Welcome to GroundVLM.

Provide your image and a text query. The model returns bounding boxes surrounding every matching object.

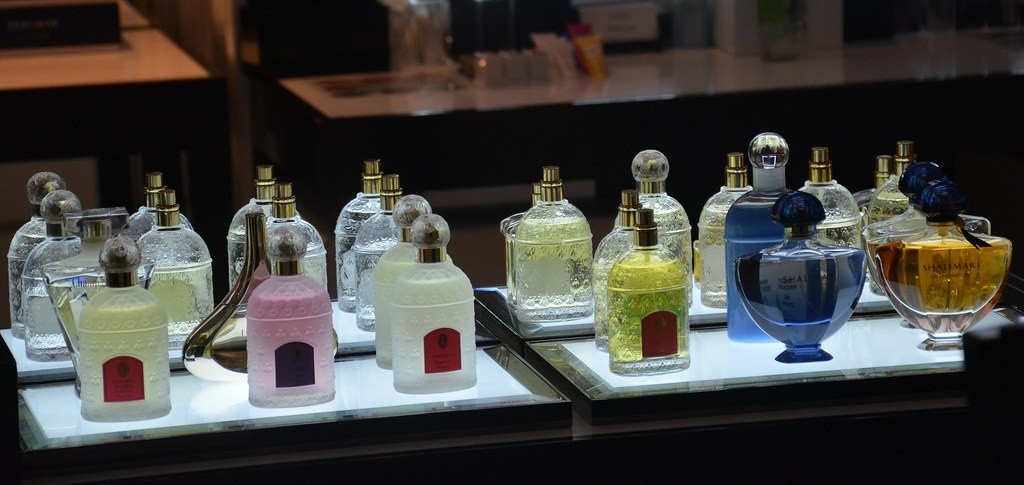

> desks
[0,275,1024,485]
[276,28,1024,234]
[0,0,234,284]
